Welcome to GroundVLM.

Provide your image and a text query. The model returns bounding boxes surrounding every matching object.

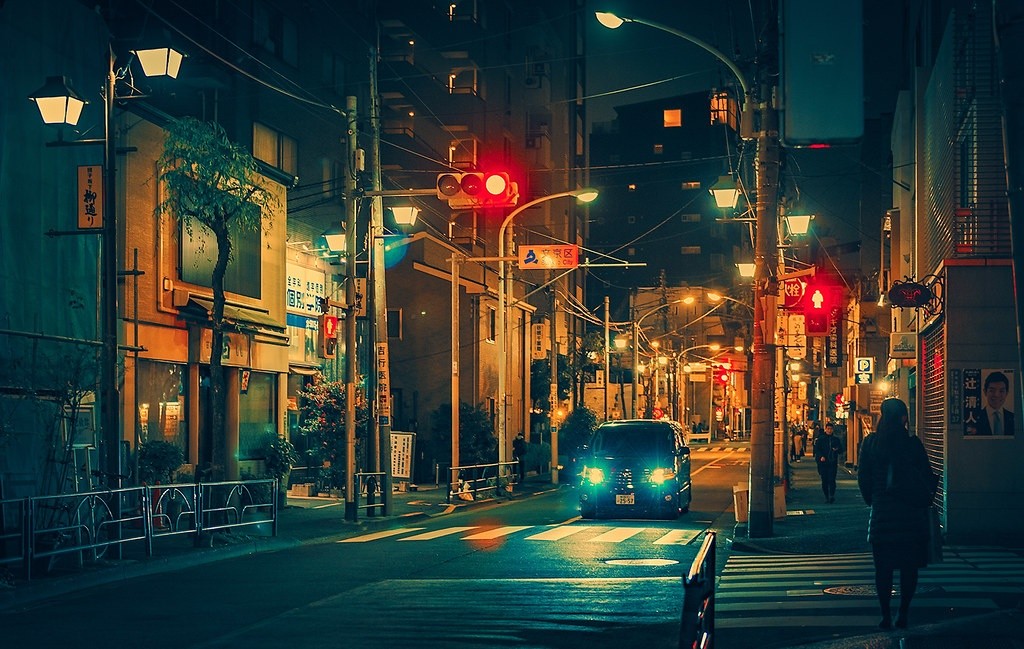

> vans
[578,416,693,518]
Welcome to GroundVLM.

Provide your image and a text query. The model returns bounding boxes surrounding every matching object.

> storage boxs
[733,481,787,521]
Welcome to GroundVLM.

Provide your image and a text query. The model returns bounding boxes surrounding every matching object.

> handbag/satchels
[926,504,943,565]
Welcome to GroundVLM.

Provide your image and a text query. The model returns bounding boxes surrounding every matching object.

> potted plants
[140,439,185,524]
[266,431,298,507]
[242,472,258,513]
[259,472,273,511]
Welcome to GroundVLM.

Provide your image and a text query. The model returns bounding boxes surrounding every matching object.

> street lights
[598,6,815,536]
[23,0,189,565]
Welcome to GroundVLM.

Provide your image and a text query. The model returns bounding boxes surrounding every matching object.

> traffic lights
[316,314,338,359]
[806,284,830,339]
[436,172,518,207]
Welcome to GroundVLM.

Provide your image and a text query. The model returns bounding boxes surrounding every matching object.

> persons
[816,422,843,505]
[691,421,697,442]
[512,432,528,481]
[696,423,703,443]
[980,372,1014,436]
[856,398,939,633]
[787,421,823,463]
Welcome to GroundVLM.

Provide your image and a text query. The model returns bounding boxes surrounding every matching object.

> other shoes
[830,497,835,503]
[895,616,907,627]
[878,617,892,628]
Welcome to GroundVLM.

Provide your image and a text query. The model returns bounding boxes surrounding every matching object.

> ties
[993,411,1003,436]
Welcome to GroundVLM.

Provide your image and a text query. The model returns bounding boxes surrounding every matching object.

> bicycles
[26,471,128,564]
